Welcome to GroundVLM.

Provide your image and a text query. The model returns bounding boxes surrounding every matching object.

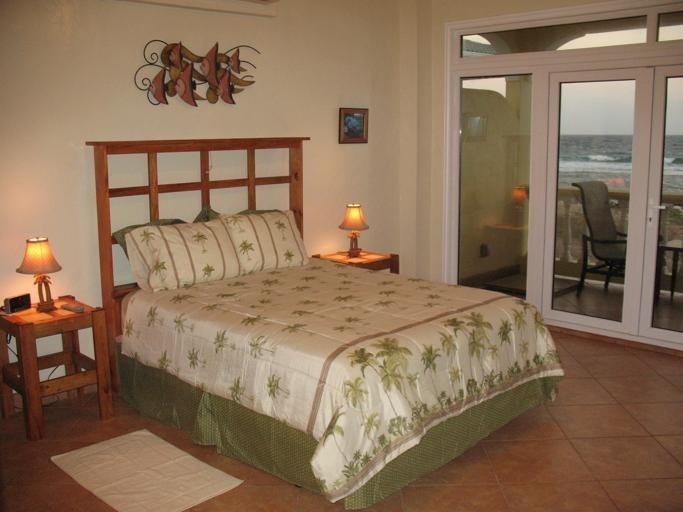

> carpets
[50,428,246,512]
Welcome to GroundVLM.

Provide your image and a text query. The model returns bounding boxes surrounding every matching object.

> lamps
[510,184,527,226]
[339,204,370,258]
[16,236,62,312]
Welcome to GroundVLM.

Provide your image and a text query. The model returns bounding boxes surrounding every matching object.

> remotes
[60,302,84,313]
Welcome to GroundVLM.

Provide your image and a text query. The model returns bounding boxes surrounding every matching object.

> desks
[659,240,683,304]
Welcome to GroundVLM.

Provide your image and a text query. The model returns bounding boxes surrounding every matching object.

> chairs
[113,206,310,295]
[572,181,663,305]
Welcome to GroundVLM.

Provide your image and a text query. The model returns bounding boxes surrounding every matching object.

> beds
[84,137,566,512]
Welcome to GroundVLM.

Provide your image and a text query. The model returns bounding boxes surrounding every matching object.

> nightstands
[312,250,399,275]
[0,295,114,441]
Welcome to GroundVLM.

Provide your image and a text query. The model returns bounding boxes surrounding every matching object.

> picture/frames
[339,107,368,144]
